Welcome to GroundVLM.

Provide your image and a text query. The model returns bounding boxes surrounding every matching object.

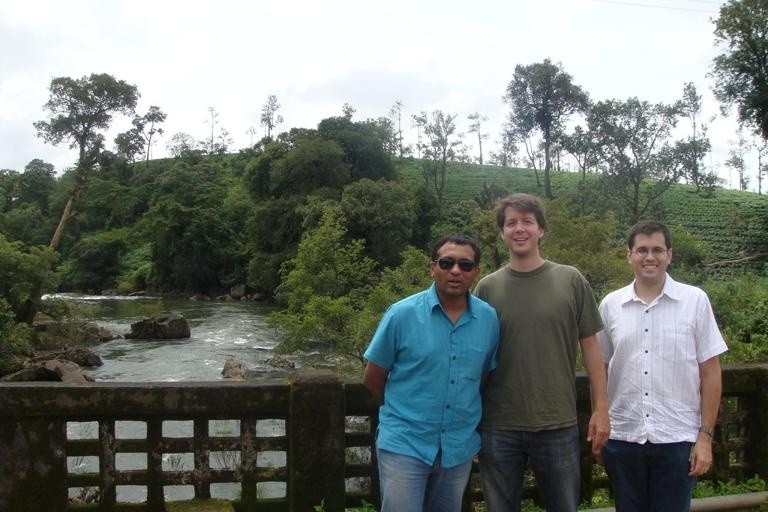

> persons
[590,220,728,510]
[360,235,504,512]
[467,189,612,512]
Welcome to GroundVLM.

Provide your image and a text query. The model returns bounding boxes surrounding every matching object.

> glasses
[632,247,669,255]
[434,257,478,272]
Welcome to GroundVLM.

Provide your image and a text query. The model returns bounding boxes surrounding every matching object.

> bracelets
[698,425,715,437]
